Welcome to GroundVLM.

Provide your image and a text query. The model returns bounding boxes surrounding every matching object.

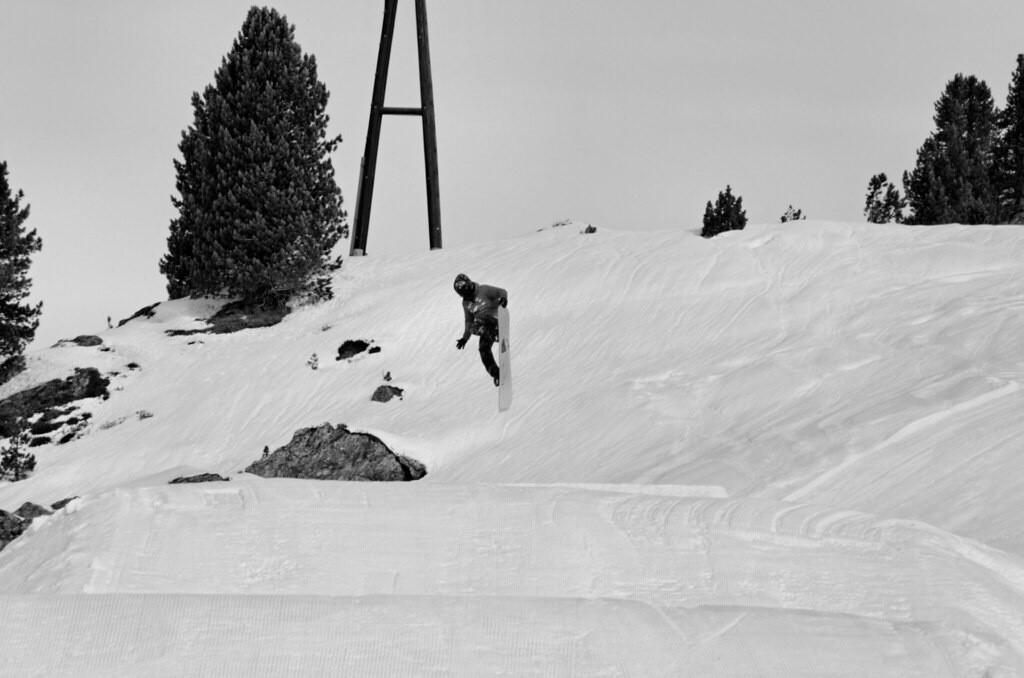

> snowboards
[498,303,514,413]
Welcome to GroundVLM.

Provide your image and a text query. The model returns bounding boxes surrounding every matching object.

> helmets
[454,274,473,298]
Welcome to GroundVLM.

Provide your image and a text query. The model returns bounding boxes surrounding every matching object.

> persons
[454,274,508,386]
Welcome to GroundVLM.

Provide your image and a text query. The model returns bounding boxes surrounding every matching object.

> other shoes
[493,369,499,386]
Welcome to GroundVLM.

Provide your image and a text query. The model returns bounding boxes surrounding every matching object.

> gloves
[456,339,466,349]
[499,297,507,308]
